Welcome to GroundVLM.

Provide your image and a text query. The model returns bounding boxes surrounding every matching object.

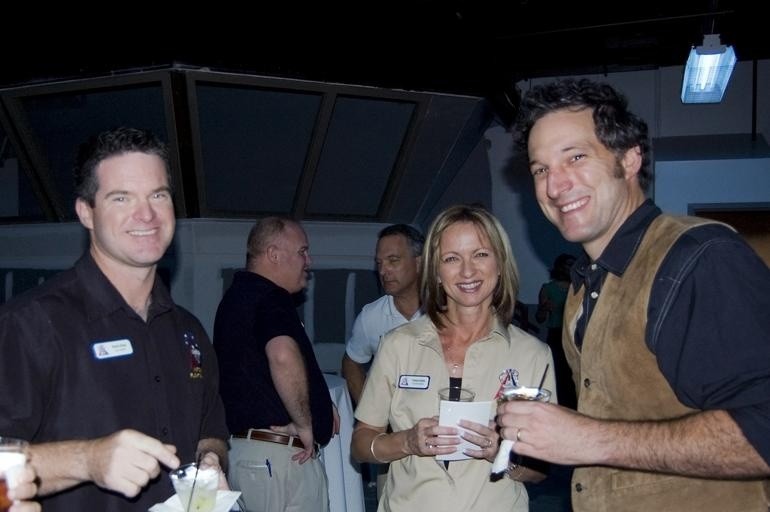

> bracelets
[505,455,521,475]
[370,432,391,464]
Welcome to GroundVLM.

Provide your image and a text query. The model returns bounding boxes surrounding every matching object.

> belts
[232,427,319,456]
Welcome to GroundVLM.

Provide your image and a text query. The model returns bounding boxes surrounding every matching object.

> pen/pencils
[266,458,272,477]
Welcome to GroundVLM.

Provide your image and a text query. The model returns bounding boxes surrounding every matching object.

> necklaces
[443,328,479,373]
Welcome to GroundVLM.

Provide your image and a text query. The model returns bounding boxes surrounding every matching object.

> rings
[517,427,522,441]
[425,438,428,447]
[429,437,432,449]
[488,439,491,448]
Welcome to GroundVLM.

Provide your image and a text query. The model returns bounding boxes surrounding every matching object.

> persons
[535,253,579,412]
[344,205,556,511]
[212,216,341,512]
[0,126,231,512]
[496,76,770,511]
[0,442,43,512]
[340,223,430,505]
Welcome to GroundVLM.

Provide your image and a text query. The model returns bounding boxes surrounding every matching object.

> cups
[498,386,553,406]
[0,435,31,512]
[168,462,221,512]
[436,386,476,418]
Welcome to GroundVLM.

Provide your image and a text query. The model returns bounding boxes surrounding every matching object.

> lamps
[680,34,737,104]
